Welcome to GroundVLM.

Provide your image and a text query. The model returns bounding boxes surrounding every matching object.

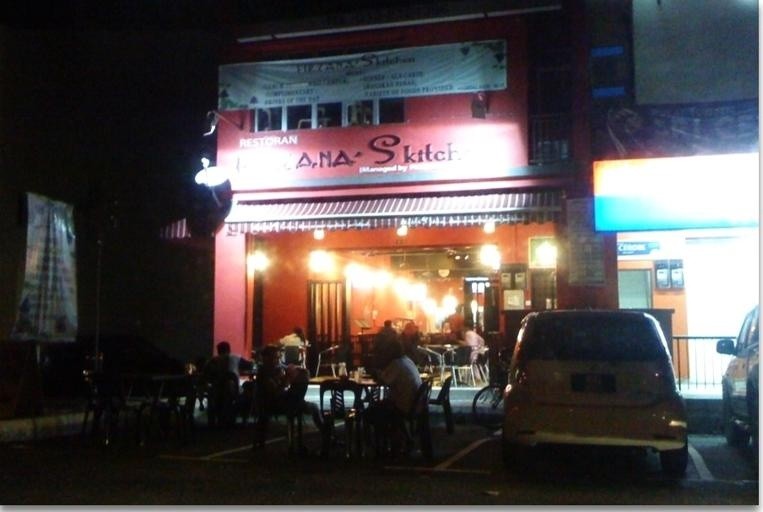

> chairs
[80,348,205,445]
[207,347,310,447]
[319,375,456,457]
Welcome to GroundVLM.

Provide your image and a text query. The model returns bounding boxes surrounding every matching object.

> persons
[377,304,486,369]
[249,343,288,386]
[200,340,253,414]
[278,328,311,364]
[282,344,305,393]
[360,319,425,468]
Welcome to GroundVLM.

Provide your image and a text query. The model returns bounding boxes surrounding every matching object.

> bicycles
[474,331,511,425]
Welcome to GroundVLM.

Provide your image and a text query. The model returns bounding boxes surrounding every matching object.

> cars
[41,333,196,400]
[717,307,759,450]
[499,310,687,471]
